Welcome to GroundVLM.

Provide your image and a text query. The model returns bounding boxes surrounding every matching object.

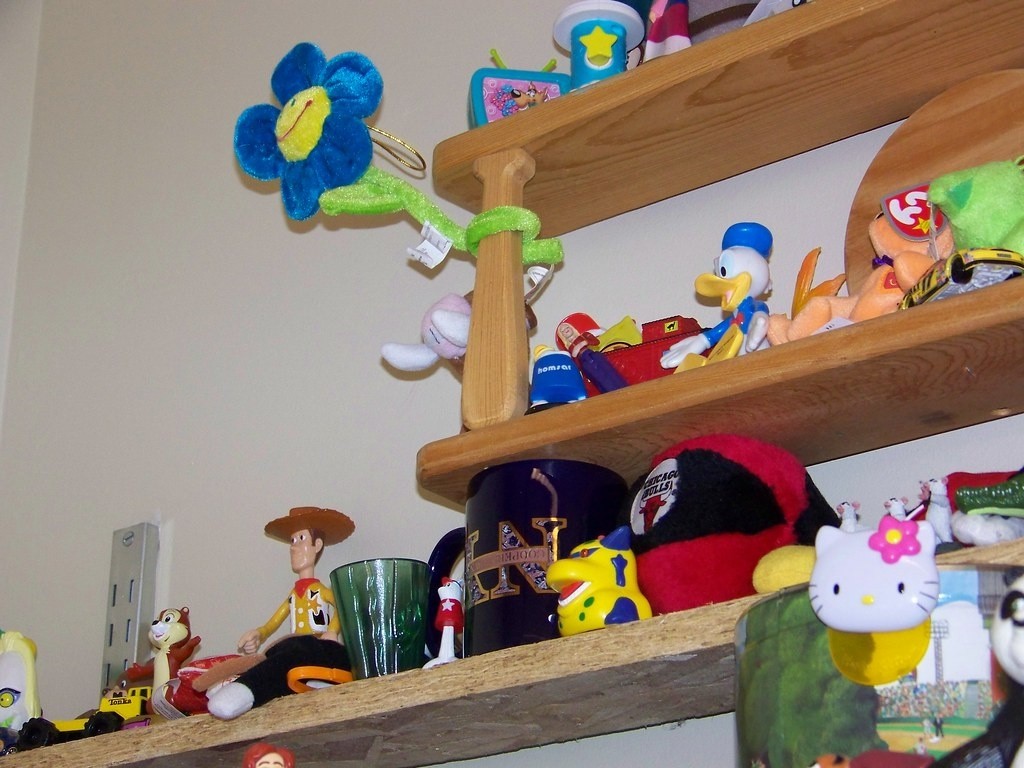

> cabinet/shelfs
[416,0,1024,519]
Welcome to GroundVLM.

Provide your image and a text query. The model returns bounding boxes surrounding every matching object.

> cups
[329,557,437,682]
[423,459,632,660]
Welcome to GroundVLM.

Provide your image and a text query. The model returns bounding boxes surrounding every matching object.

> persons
[209,502,356,718]
[244,742,296,768]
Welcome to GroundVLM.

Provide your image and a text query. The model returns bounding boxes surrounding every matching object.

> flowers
[868,514,921,563]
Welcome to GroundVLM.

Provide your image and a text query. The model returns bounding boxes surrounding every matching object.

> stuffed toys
[639,425,840,619]
[763,159,1024,347]
